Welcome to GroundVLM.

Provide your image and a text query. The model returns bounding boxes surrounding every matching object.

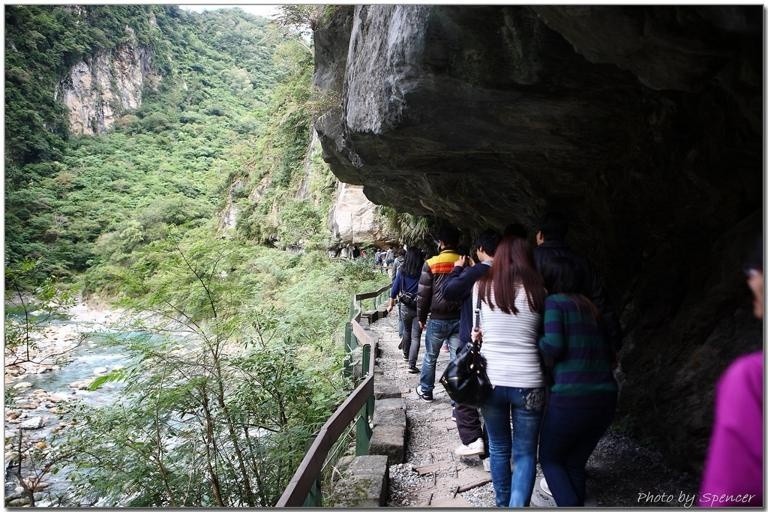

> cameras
[462,256,470,267]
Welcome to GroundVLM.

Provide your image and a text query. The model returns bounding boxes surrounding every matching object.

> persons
[261,218,623,509]
[691,246,763,508]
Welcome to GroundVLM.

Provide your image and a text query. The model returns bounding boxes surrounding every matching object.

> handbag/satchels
[398,291,417,309]
[439,342,493,406]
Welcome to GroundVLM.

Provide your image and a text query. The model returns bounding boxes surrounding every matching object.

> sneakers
[455,438,485,455]
[482,457,491,471]
[403,355,409,361]
[440,344,449,351]
[407,367,420,373]
[415,384,433,403]
